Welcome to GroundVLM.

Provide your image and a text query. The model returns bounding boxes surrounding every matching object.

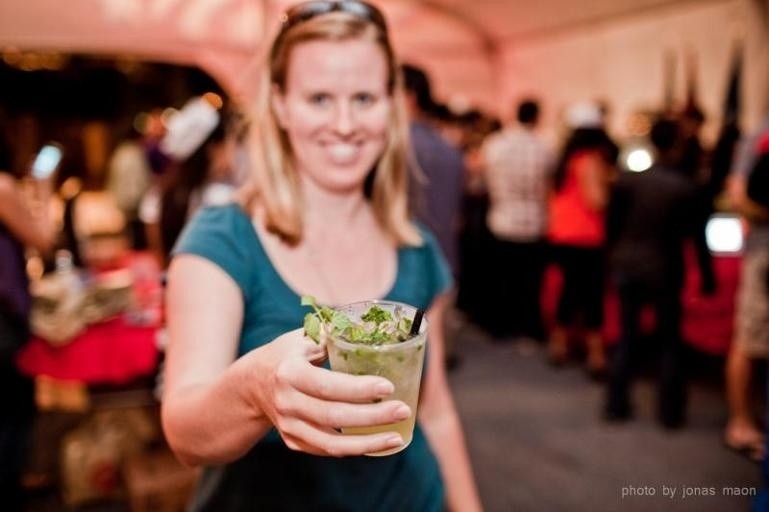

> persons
[2,52,768,510]
[156,3,486,511]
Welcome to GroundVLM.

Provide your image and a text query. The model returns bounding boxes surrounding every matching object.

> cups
[318,298,430,458]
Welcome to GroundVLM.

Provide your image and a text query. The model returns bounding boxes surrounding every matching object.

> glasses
[267,0,391,54]
